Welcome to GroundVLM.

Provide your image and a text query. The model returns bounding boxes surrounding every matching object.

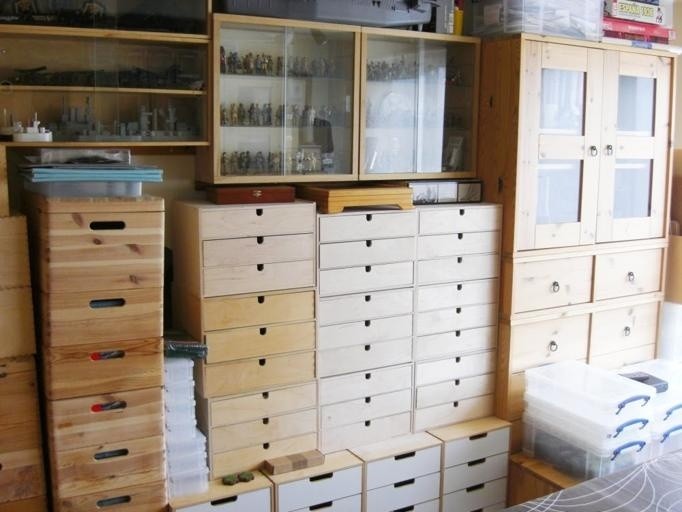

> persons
[221,102,336,127]
[220,46,336,79]
[367,55,463,83]
[222,150,317,175]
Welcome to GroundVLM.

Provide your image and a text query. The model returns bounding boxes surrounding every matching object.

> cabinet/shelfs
[196,11,361,187]
[493,297,664,424]
[0,29,212,147]
[0,0,211,38]
[498,243,669,321]
[358,24,481,182]
[477,30,678,256]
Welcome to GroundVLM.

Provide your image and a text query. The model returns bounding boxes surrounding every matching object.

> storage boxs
[47,386,164,454]
[55,480,168,512]
[180,287,317,398]
[207,187,295,204]
[472,0,602,42]
[440,451,508,493]
[169,473,275,512]
[397,499,439,512]
[293,493,361,512]
[426,415,512,469]
[418,203,504,286]
[51,434,166,498]
[347,432,441,491]
[38,196,166,292]
[521,359,681,483]
[481,502,506,512]
[440,478,507,512]
[260,449,363,512]
[177,202,316,298]
[319,363,414,454]
[45,339,163,399]
[413,276,501,362]
[43,289,169,347]
[316,209,417,297]
[362,473,440,511]
[317,288,414,382]
[197,379,318,481]
[412,349,497,432]
